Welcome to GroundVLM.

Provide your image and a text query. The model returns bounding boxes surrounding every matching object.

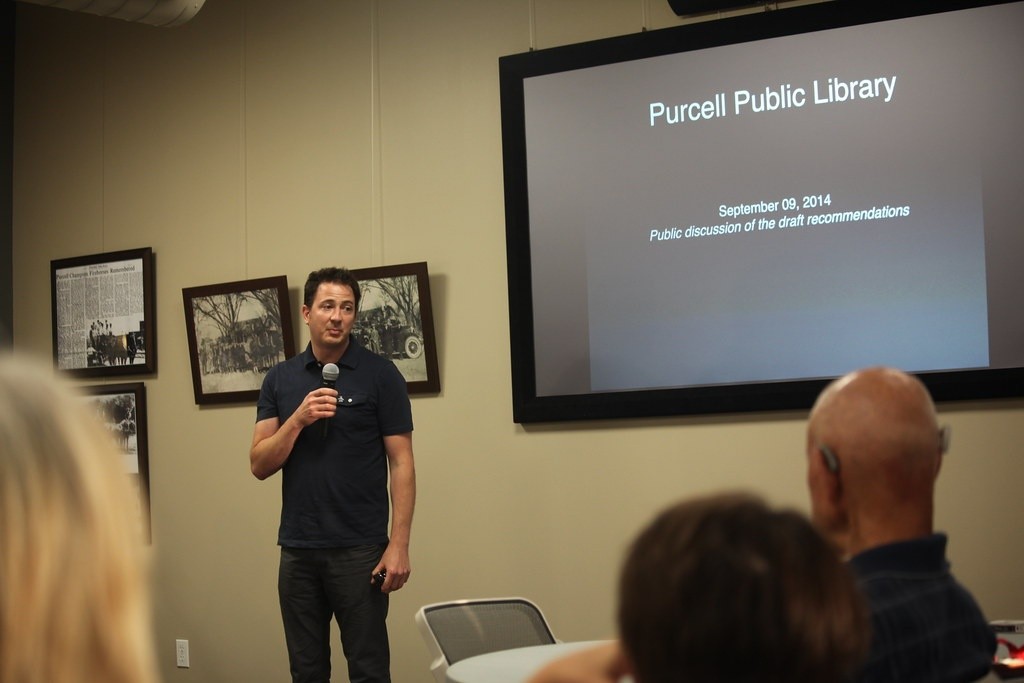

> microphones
[321,363,339,439]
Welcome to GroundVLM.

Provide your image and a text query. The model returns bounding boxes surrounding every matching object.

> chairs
[415,597,563,683]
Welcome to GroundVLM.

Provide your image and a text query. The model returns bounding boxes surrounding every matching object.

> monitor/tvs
[499,0,1024,424]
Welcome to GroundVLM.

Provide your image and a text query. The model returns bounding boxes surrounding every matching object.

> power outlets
[176,639,189,668]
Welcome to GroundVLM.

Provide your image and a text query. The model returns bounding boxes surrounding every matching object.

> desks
[447,639,635,683]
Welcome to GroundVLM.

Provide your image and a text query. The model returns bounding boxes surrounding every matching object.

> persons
[0,350,163,683]
[527,492,873,683]
[248,267,417,683]
[356,319,404,361]
[199,338,226,375]
[807,369,996,683]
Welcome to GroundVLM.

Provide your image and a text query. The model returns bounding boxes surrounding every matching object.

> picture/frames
[350,261,440,394]
[50,247,158,378]
[79,382,152,547]
[183,275,295,404]
